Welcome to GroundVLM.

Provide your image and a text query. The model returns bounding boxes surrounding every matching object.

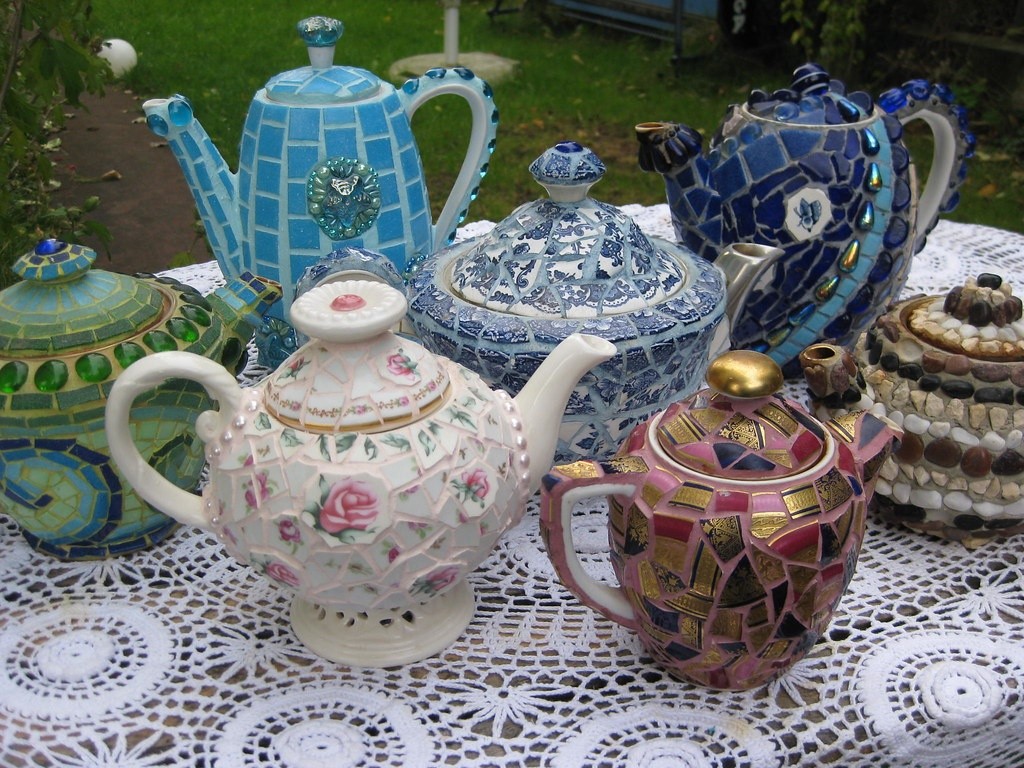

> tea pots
[633,62,974,385]
[799,272,1023,543]
[105,279,619,670]
[0,236,284,563]
[141,15,502,371]
[290,141,787,492]
[539,349,906,693]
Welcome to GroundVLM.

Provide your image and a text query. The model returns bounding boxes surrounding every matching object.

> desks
[0,204,1024,767]
